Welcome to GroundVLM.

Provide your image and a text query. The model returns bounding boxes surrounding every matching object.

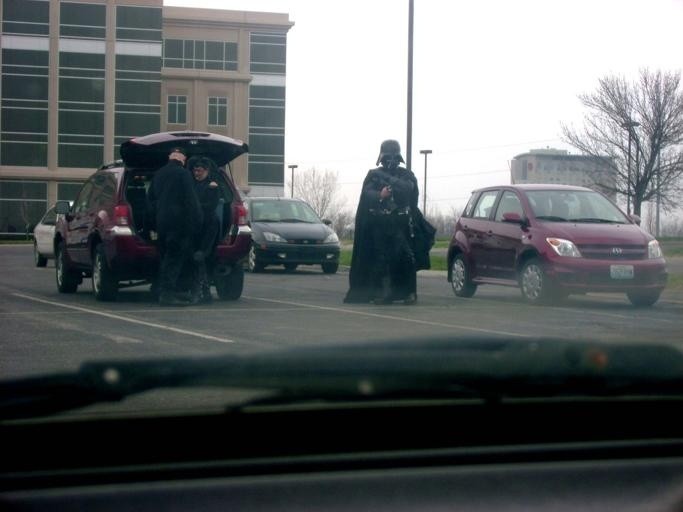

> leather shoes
[158,294,189,306]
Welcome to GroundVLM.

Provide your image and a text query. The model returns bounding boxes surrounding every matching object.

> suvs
[53,131,251,301]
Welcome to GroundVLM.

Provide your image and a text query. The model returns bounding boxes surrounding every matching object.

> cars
[33,200,73,267]
[447,184,669,307]
[241,197,340,273]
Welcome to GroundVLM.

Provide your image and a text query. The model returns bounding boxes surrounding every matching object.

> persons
[146,144,202,307]
[179,154,233,307]
[343,139,436,307]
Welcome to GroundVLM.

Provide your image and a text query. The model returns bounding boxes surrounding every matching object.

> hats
[169,147,186,154]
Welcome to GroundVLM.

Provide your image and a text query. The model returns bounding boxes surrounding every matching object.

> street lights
[417,148,433,221]
[287,163,298,197]
[619,121,640,216]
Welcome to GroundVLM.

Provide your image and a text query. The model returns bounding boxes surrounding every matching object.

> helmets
[376,141,404,165]
